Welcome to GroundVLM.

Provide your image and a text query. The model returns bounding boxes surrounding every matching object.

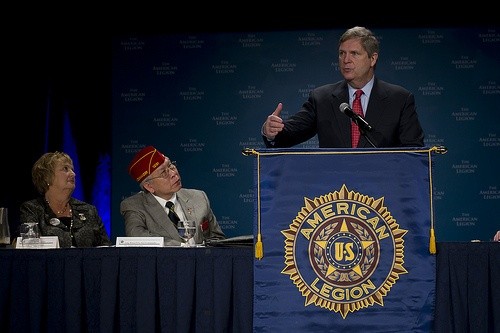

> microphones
[340,103,373,132]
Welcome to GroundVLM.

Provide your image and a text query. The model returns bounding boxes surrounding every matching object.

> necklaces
[48,199,73,237]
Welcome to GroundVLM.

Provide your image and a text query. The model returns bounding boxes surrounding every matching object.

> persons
[491,229,500,242]
[262,27,425,148]
[18,152,113,248]
[120,146,227,246]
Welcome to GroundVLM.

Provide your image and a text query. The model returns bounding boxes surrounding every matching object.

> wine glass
[177,221,196,247]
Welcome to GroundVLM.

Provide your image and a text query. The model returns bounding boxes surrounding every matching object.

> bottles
[0,207,10,248]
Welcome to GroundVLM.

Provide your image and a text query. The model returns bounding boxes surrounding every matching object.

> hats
[127,145,165,186]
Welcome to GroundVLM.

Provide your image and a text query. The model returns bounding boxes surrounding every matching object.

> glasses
[143,161,176,188]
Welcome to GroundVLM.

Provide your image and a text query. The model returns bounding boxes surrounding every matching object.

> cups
[20,223,40,249]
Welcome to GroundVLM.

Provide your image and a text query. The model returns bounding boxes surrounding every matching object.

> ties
[165,201,186,233]
[352,90,365,148]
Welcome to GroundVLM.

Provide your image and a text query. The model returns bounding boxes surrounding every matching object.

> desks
[433,241,500,333]
[0,247,254,333]
